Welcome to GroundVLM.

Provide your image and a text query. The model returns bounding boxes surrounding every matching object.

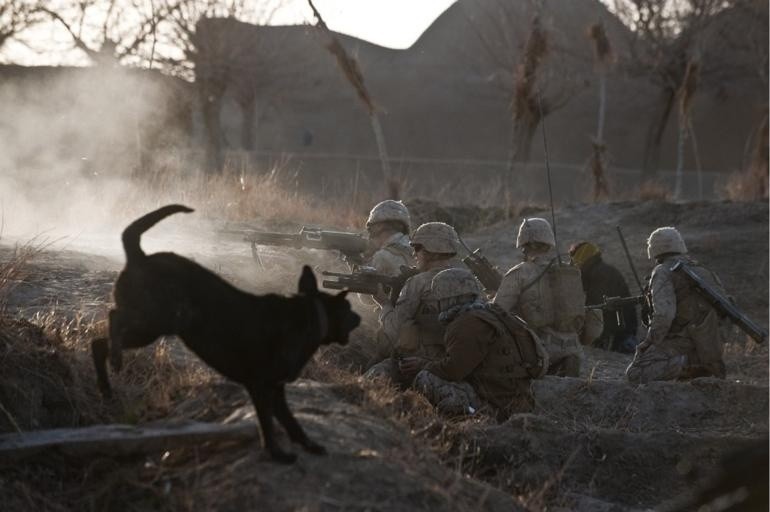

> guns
[219,226,369,272]
[322,264,419,308]
[459,236,503,299]
[585,294,647,326]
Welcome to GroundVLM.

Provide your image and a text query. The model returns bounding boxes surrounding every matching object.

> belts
[538,329,577,347]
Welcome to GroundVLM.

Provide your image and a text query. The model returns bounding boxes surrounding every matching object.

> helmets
[366,199,410,232]
[409,222,458,254]
[516,218,555,249]
[430,267,480,301]
[648,227,689,261]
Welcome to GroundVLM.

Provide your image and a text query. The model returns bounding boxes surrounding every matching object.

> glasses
[414,245,423,252]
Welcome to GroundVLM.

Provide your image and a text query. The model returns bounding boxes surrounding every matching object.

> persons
[567,242,638,354]
[370,222,486,378]
[398,267,535,425]
[624,224,727,386]
[331,198,417,317]
[483,217,587,379]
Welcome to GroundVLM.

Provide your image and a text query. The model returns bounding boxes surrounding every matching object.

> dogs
[88,202,362,465]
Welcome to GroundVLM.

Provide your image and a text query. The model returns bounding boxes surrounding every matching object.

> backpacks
[489,303,549,380]
[520,257,585,332]
[687,268,736,344]
